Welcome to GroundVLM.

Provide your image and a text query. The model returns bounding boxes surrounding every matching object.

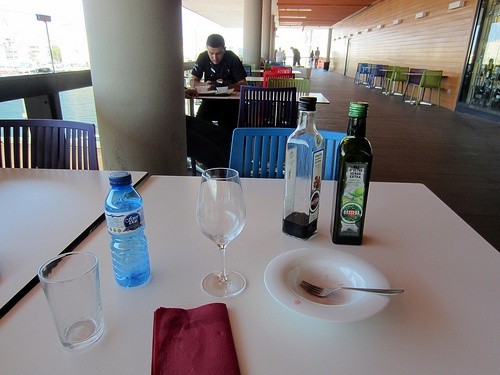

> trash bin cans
[324,62,329,71]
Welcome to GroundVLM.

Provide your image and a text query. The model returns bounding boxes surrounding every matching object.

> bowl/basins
[196,86,209,92]
[216,87,228,92]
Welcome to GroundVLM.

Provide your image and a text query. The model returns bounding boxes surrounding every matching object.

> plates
[198,93,233,96]
[264,248,391,322]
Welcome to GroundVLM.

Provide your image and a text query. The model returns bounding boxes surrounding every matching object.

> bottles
[282,97,325,242]
[330,102,374,246]
[104,172,151,288]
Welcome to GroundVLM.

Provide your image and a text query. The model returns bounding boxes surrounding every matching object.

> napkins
[151,302,241,375]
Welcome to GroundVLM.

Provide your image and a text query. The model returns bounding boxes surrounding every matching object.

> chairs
[226,64,347,181]
[354,61,443,108]
[0,119,99,170]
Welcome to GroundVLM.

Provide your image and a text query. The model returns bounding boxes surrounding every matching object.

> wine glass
[195,167,247,298]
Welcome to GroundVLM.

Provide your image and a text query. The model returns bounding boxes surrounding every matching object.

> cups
[38,252,105,349]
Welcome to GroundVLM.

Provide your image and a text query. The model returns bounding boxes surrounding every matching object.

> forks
[298,281,404,298]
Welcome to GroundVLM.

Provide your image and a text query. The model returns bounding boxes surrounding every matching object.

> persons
[309,50,314,63]
[190,34,248,129]
[273,47,286,66]
[479,59,495,93]
[290,47,301,67]
[315,46,320,69]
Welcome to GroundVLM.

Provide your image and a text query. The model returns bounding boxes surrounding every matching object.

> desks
[0,168,500,375]
[250,69,302,74]
[401,73,448,106]
[379,69,403,95]
[184,86,330,118]
[247,76,303,81]
